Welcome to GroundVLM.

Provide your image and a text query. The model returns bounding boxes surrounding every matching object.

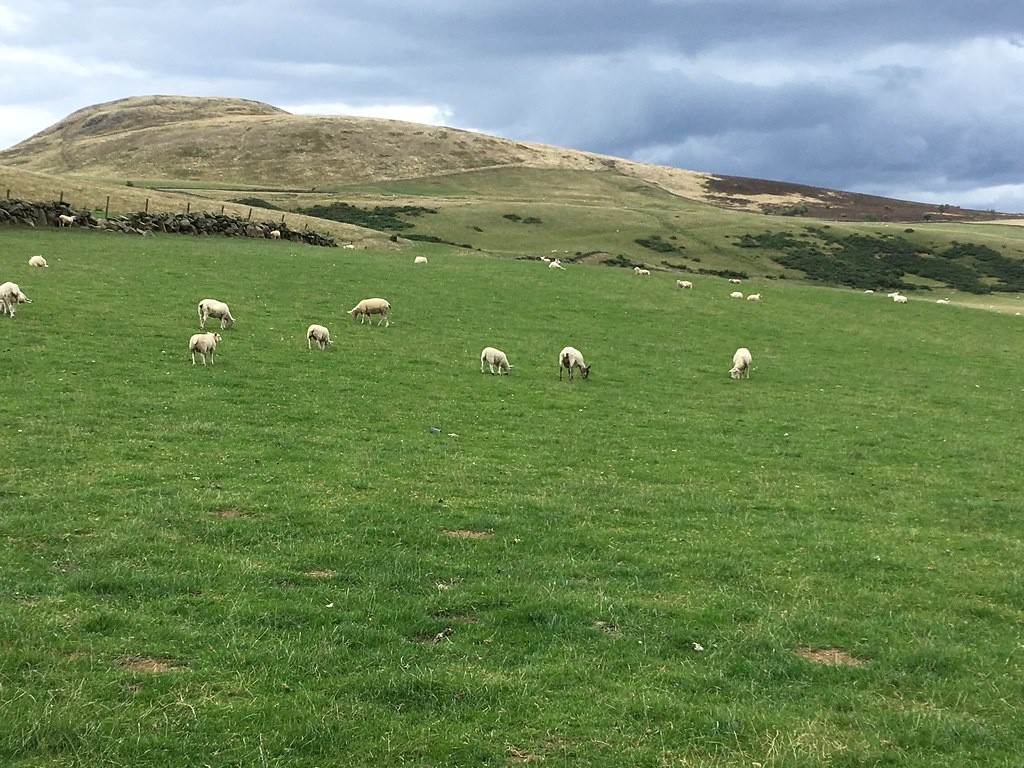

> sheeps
[729,278,741,284]
[58,214,76,228]
[936,297,950,304]
[306,323,333,351]
[28,254,50,268]
[198,298,236,332]
[728,348,752,379]
[189,330,223,365]
[480,346,514,376]
[729,291,743,299]
[347,297,392,329]
[634,266,650,276]
[864,289,875,294]
[746,292,763,301]
[342,244,356,249]
[270,230,281,240]
[676,279,693,290]
[559,346,591,382]
[540,256,566,271]
[0,282,33,319]
[413,256,427,264]
[888,291,908,304]
